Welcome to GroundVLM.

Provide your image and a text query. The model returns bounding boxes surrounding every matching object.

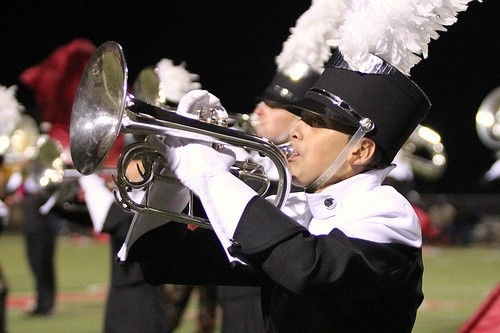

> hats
[249,0,357,107]
[284,1,483,165]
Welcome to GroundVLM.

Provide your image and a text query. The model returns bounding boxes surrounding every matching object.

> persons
[1,42,205,333]
[111,0,483,333]
[194,0,379,333]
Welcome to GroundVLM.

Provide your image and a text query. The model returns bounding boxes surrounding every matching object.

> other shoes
[22,304,55,317]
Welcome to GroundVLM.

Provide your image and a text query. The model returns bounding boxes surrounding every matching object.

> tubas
[69,41,296,231]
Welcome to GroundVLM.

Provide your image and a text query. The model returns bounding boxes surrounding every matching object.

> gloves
[116,87,229,263]
[77,174,115,236]
[163,136,258,267]
